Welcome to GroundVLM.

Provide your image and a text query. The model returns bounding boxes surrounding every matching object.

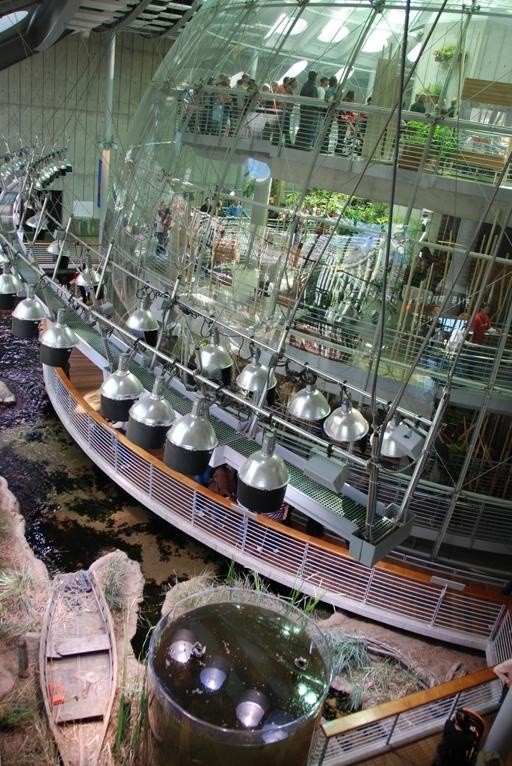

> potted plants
[399,119,446,172]
[414,83,443,104]
[433,45,470,69]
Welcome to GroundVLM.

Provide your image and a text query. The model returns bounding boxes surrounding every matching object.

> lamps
[286,374,331,419]
[235,351,278,391]
[125,288,160,331]
[149,295,178,324]
[370,413,409,459]
[101,352,144,422]
[126,374,176,450]
[164,396,219,475]
[391,418,429,460]
[0,143,114,366]
[236,429,290,514]
[324,393,370,442]
[193,326,234,369]
[304,447,348,493]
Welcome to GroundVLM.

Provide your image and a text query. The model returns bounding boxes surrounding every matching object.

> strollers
[342,114,366,157]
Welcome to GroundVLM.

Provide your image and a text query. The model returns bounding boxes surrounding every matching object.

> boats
[39,569,118,766]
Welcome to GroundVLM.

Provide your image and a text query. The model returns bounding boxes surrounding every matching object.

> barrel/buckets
[143,585,336,766]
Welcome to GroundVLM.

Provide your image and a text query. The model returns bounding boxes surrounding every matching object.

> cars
[461,133,512,178]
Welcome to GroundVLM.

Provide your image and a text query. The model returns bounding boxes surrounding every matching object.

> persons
[200,197,248,218]
[469,303,491,377]
[420,304,442,363]
[403,246,433,288]
[410,95,427,113]
[195,463,290,553]
[435,423,455,461]
[178,71,372,157]
[154,208,173,255]
[448,100,456,117]
